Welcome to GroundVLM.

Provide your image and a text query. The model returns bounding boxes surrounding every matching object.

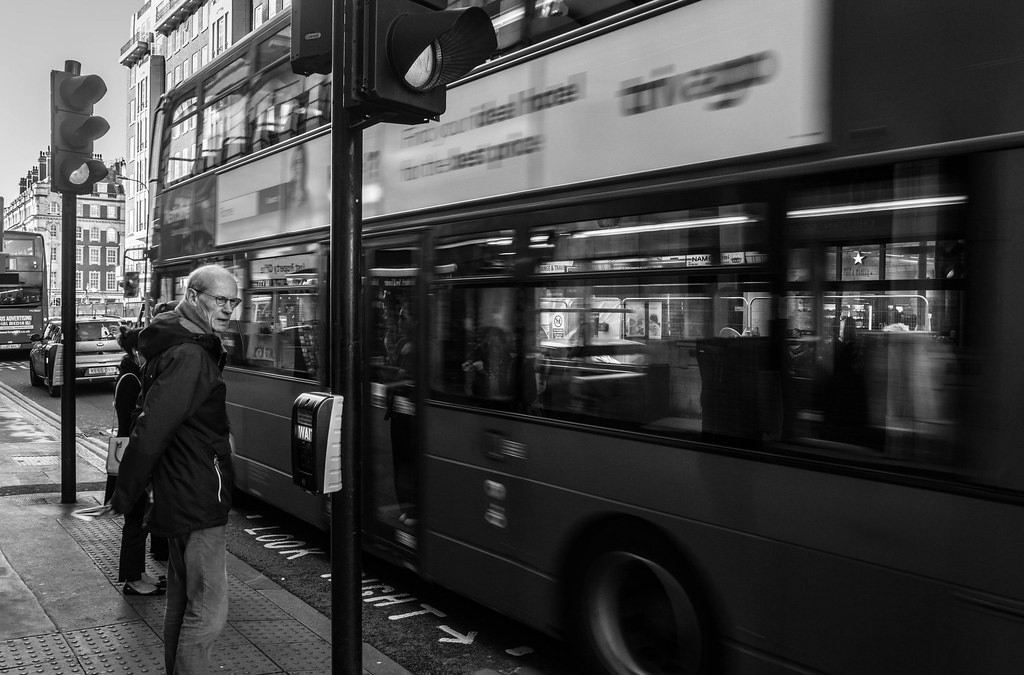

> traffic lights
[359,0,499,116]
[50,70,108,194]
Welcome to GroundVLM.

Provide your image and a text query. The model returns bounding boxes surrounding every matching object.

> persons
[106,264,241,675]
[149,300,181,565]
[102,325,168,597]
[275,146,311,229]
[375,288,515,405]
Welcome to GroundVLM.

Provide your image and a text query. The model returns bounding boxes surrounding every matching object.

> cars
[30,315,132,397]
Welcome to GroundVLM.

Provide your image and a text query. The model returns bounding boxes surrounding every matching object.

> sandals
[124,571,166,595]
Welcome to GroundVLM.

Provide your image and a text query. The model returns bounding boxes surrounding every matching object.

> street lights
[117,177,150,293]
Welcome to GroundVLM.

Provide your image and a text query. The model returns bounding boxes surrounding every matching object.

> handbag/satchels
[106,435,130,475]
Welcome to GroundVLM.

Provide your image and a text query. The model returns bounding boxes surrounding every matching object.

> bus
[119,0,1024,675]
[0,230,50,355]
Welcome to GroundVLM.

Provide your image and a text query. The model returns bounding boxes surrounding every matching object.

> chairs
[284,104,329,139]
[190,149,221,177]
[247,123,285,155]
[220,137,251,165]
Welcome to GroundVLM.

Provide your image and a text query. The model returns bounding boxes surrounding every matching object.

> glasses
[190,285,242,307]
